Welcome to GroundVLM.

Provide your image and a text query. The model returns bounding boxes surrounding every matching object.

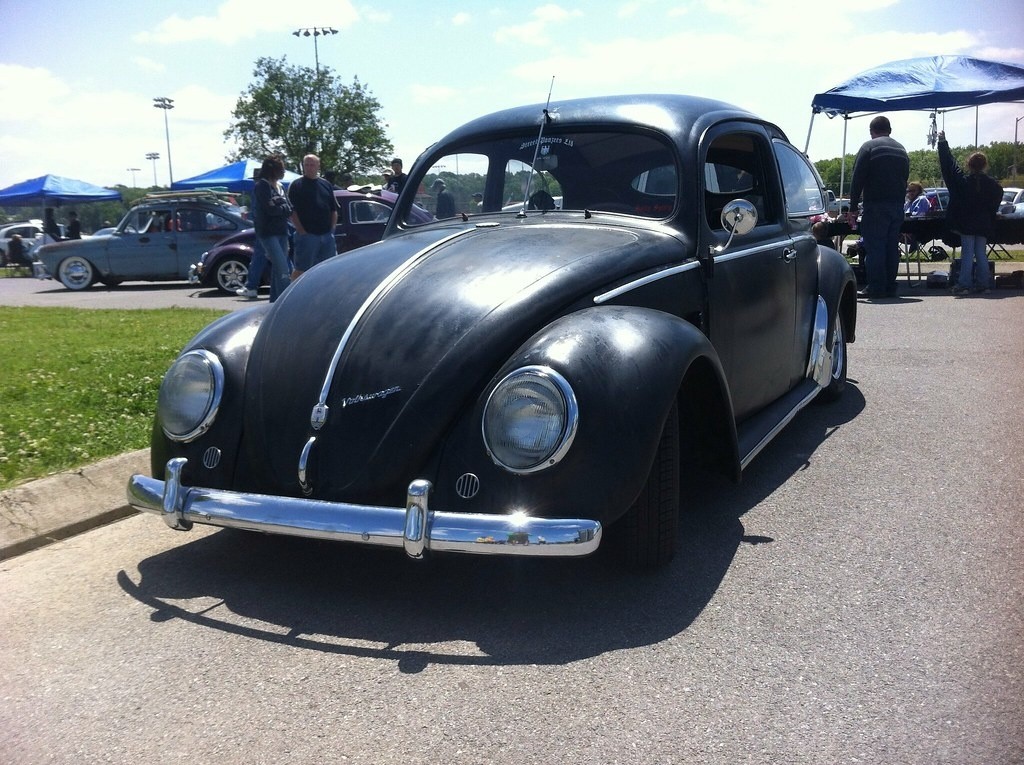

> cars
[923,188,948,215]
[33,197,256,293]
[996,187,1024,220]
[128,93,855,583]
[805,189,850,214]
[189,189,439,296]
[0,222,92,268]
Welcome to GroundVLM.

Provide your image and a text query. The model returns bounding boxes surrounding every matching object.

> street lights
[152,96,175,184]
[145,153,159,186]
[292,26,339,139]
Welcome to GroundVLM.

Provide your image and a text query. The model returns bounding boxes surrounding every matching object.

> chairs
[167,218,183,232]
[6,250,29,278]
[985,205,1016,260]
[896,216,936,261]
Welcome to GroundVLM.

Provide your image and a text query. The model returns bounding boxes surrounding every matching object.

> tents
[803,56,1024,253]
[168,158,303,204]
[0,174,125,244]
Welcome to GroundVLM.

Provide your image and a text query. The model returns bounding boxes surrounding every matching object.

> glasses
[842,213,848,215]
[908,189,918,193]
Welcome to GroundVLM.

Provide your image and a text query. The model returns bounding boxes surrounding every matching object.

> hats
[472,192,483,198]
[431,178,445,189]
[381,168,394,176]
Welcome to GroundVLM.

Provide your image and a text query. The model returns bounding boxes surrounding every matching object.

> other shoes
[235,286,258,298]
[950,285,969,295]
[856,284,886,298]
[974,286,992,294]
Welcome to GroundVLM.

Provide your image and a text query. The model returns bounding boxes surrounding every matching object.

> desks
[813,213,1022,288]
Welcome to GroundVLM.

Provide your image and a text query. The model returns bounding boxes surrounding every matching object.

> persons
[7,234,34,276]
[810,181,931,278]
[65,211,82,240]
[236,154,408,304]
[472,192,484,212]
[148,210,207,232]
[430,179,456,221]
[842,113,908,297]
[936,130,1005,294]
[44,208,61,240]
